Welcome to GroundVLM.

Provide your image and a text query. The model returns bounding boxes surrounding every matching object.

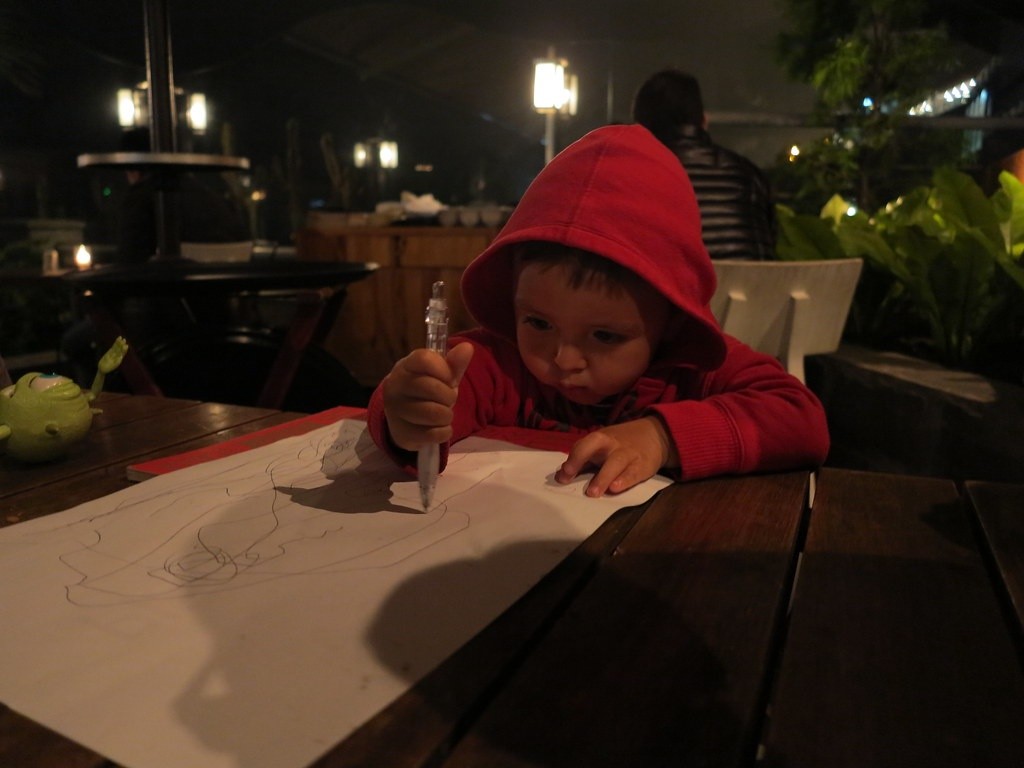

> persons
[366,125,829,497]
[633,71,782,261]
[83,128,235,398]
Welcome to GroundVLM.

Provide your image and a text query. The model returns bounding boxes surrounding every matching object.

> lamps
[114,79,147,133]
[530,45,579,163]
[174,79,209,137]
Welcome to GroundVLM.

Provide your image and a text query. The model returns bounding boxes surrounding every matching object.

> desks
[0,390,1023,768]
[0,257,374,408]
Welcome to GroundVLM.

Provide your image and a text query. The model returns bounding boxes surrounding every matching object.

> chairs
[712,256,863,508]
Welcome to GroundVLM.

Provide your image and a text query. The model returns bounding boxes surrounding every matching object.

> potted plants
[771,160,1023,482]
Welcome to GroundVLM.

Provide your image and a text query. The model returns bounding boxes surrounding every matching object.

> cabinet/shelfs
[296,224,497,387]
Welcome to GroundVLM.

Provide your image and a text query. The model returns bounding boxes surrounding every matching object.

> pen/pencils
[415,280,448,511]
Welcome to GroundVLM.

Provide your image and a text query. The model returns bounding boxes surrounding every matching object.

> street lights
[533,59,580,168]
